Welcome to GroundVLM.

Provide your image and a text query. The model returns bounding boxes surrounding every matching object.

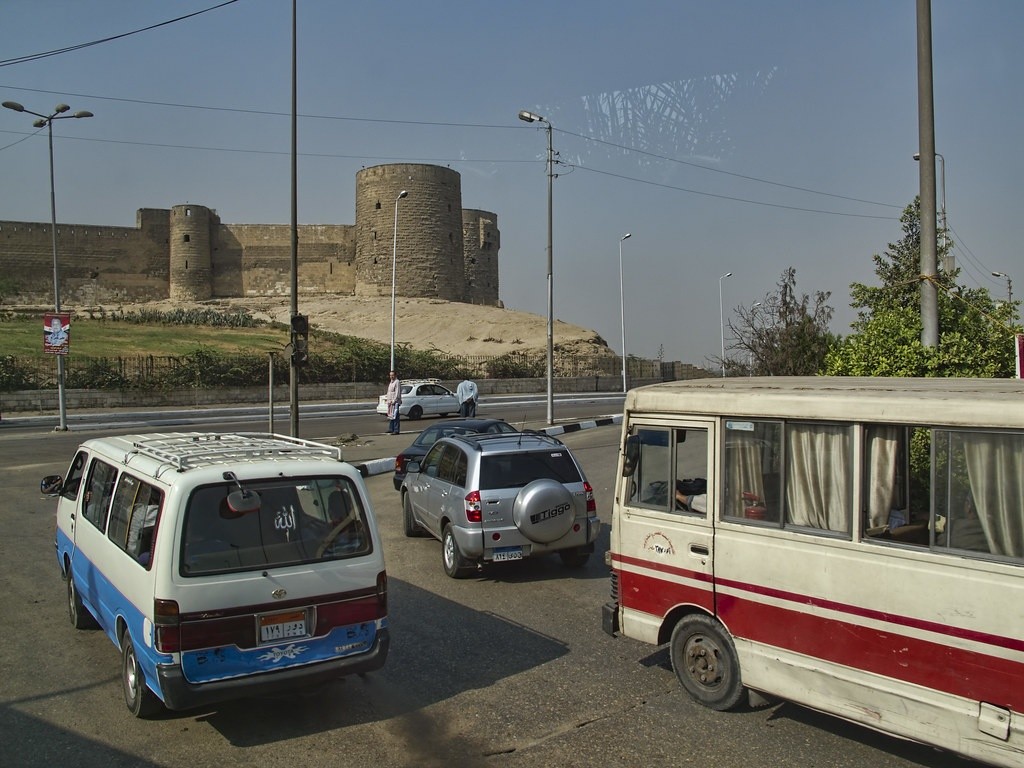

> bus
[41,431,391,718]
[603,376,1024,768]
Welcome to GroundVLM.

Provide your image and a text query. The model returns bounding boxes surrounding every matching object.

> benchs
[866,509,939,544]
[187,532,355,571]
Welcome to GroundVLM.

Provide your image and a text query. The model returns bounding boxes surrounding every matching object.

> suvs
[399,429,600,578]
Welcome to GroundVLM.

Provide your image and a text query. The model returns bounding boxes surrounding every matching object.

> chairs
[480,462,503,489]
[421,387,429,395]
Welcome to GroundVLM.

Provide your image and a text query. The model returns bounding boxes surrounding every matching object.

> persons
[457,376,478,417]
[952,490,990,554]
[319,490,360,536]
[48,317,68,346]
[385,371,401,435]
[676,490,706,514]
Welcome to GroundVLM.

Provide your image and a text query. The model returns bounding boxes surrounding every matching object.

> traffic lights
[290,315,308,367]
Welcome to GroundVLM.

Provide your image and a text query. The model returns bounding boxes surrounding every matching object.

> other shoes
[390,431,399,435]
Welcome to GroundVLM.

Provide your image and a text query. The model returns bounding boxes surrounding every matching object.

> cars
[393,420,519,492]
[377,382,478,421]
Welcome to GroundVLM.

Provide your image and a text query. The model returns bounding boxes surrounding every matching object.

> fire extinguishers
[741,491,769,520]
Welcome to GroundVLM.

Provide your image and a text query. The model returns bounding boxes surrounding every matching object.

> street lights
[391,190,408,371]
[749,303,761,376]
[620,233,632,392]
[913,152,946,258]
[2,101,95,431]
[518,110,554,425]
[992,272,1011,324]
[265,351,278,439]
[719,273,732,377]
